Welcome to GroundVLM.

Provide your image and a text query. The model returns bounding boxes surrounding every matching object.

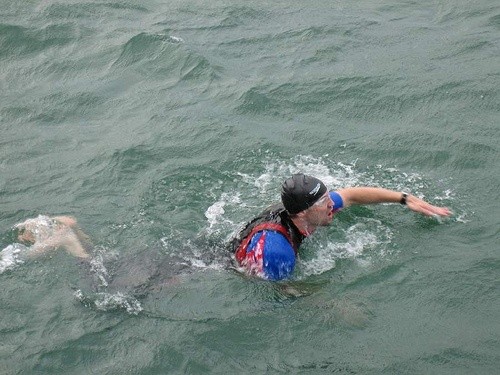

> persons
[16,173,455,297]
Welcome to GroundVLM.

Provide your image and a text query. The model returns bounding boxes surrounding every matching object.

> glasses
[311,194,331,207]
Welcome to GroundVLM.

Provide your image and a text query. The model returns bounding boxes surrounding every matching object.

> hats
[281,173,328,214]
